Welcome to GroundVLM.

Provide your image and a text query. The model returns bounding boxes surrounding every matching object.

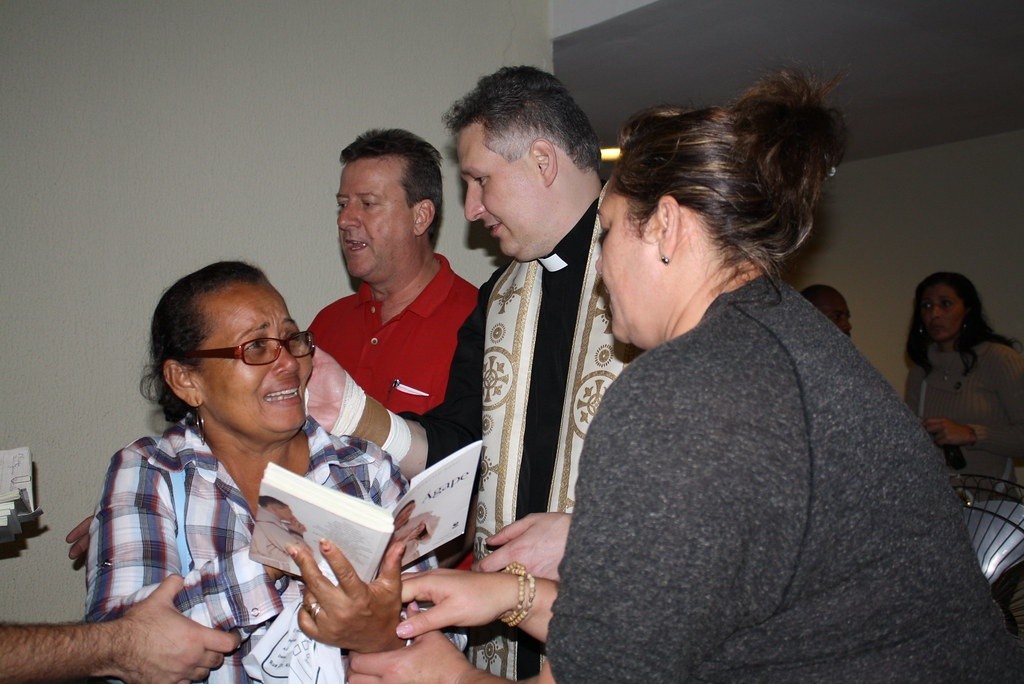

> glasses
[172,331,315,365]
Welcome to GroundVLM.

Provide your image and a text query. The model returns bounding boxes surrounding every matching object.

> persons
[249,495,315,572]
[904,272,1024,500]
[0,574,241,684]
[800,284,852,339]
[302,65,647,684]
[65,128,479,572]
[348,73,1016,684]
[88,260,465,684]
[387,500,443,567]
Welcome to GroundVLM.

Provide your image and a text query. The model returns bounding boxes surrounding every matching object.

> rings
[305,602,319,611]
[314,607,322,620]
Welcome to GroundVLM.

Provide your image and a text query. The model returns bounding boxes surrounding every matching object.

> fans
[949,473,1023,645]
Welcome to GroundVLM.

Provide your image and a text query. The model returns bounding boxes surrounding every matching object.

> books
[0,445,45,543]
[249,440,483,584]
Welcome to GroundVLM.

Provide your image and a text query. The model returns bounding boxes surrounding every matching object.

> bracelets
[501,563,537,628]
